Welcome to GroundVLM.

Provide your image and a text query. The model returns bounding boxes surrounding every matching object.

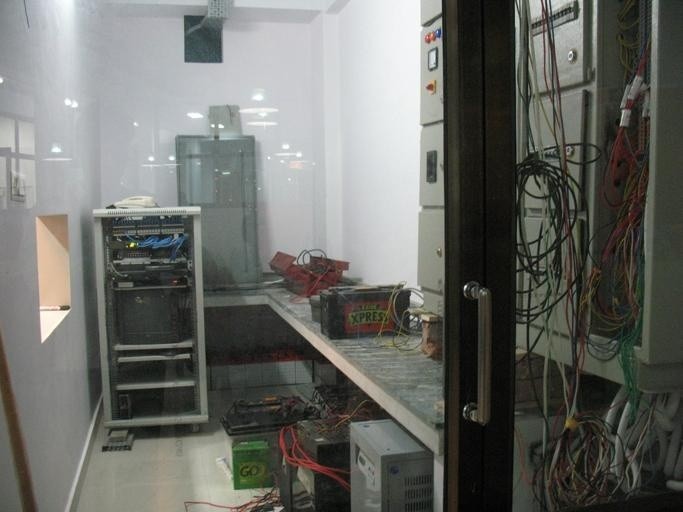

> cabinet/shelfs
[91,206,210,429]
[512,0,656,512]
[416,0,447,317]
